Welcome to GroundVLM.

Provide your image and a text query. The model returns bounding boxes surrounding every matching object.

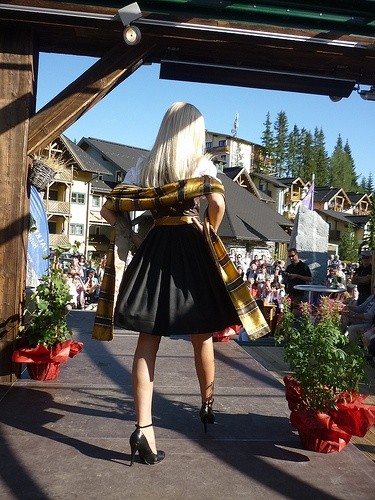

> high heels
[129,424,165,466]
[200,398,215,432]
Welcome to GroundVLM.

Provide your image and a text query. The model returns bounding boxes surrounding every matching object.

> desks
[294,285,347,314]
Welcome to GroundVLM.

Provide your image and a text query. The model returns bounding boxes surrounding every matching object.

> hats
[361,250,373,256]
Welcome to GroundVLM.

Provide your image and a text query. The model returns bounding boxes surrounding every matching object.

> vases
[283,376,375,454]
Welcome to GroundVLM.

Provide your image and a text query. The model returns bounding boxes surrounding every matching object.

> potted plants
[11,241,84,381]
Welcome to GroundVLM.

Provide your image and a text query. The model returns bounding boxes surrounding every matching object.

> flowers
[276,294,366,409]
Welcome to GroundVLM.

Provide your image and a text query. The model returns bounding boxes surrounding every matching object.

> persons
[99,101,271,467]
[326,250,375,368]
[228,250,313,337]
[56,253,106,310]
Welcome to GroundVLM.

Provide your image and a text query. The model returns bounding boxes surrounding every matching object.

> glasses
[288,253,296,258]
[362,256,370,259]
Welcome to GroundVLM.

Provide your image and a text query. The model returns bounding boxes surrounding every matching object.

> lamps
[117,2,143,45]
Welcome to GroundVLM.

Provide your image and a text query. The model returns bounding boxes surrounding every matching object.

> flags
[295,172,315,214]
